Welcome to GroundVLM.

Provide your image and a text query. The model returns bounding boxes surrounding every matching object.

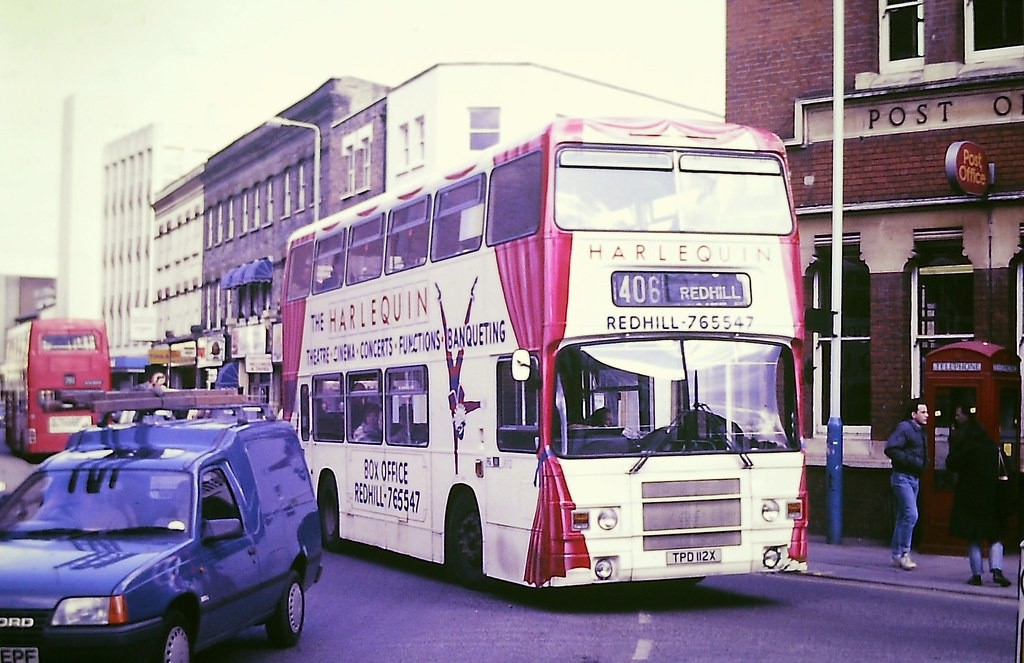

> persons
[321,255,356,290]
[389,406,421,446]
[587,405,618,427]
[943,405,1014,588]
[353,402,381,442]
[883,398,929,569]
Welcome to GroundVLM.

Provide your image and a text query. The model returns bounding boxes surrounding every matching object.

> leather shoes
[891,553,902,563]
[993,570,1012,588]
[968,576,983,586]
[897,554,918,568]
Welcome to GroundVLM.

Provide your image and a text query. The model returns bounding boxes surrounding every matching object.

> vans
[0,402,324,662]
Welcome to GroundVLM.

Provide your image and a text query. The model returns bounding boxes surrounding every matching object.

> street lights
[264,113,324,220]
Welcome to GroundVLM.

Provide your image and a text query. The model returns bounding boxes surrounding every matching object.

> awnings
[221,267,237,289]
[242,257,273,284]
[231,264,249,289]
[213,362,239,387]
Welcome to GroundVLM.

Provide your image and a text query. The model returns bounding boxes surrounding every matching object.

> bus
[280,116,812,608]
[0,315,117,466]
